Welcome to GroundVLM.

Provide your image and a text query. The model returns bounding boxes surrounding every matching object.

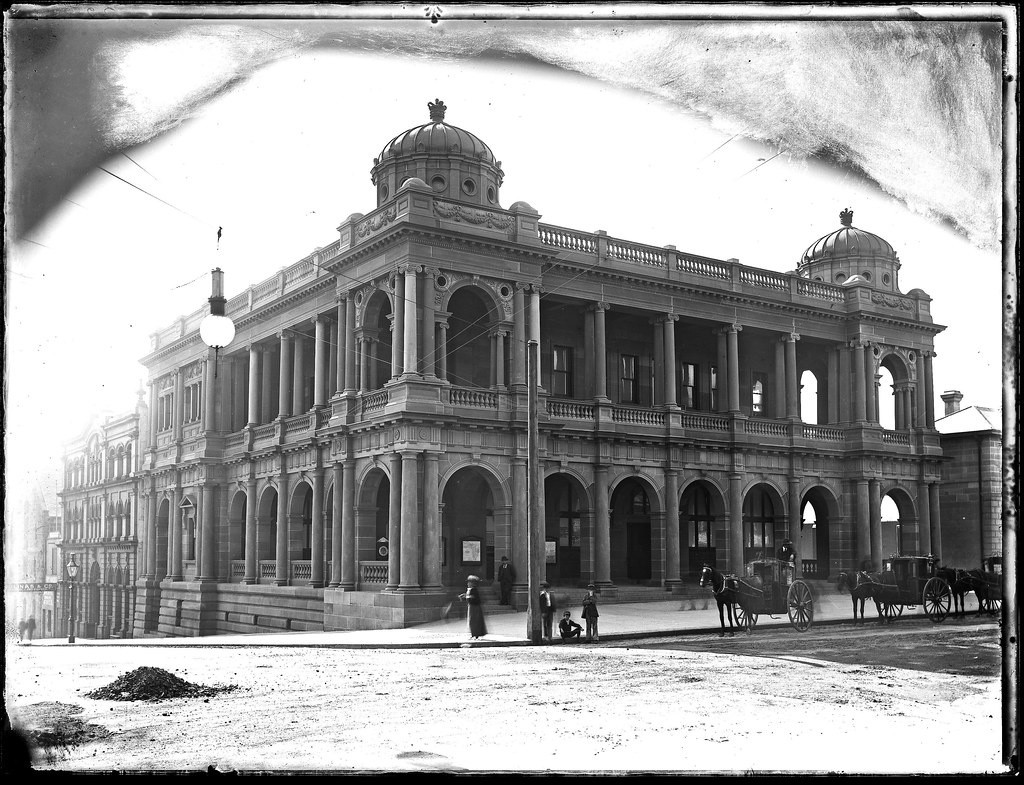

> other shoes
[468,636,479,640]
[562,642,566,645]
[578,640,581,642]
[542,636,550,642]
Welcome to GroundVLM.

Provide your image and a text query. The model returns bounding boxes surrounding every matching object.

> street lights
[66,552,82,643]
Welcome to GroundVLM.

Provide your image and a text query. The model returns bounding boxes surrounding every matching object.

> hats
[782,538,793,545]
[502,555,508,562]
[464,575,482,583]
[563,611,570,616]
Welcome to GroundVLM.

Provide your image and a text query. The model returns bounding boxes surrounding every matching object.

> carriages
[936,554,1003,619]
[699,557,817,636]
[835,551,953,624]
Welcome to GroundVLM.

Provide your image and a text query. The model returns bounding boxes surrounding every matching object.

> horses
[835,569,894,625]
[938,565,995,617]
[700,565,759,637]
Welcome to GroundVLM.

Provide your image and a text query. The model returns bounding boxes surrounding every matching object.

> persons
[457,575,488,641]
[558,611,584,645]
[539,583,557,643]
[776,539,797,563]
[18,614,36,642]
[497,556,516,606]
[580,584,599,645]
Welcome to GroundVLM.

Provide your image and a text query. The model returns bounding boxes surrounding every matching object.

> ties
[785,547,786,551]
